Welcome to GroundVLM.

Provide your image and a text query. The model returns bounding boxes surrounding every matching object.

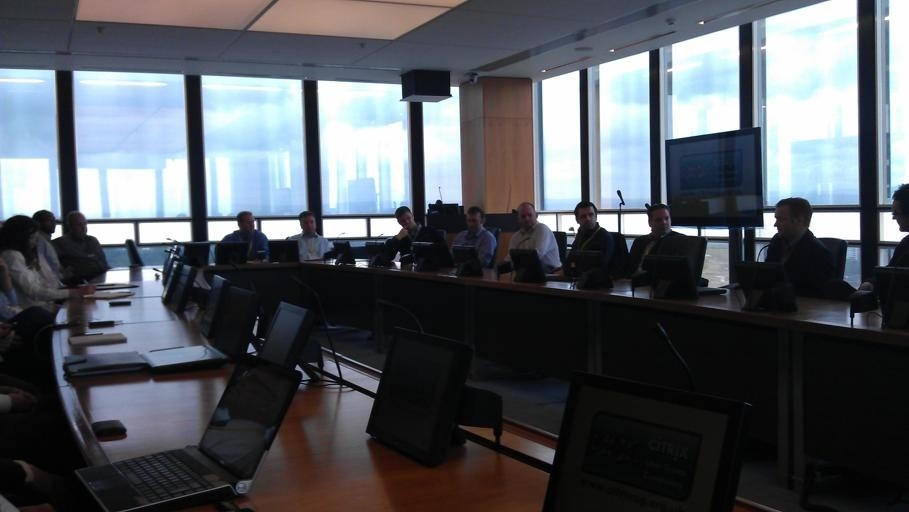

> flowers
[645,203,650,209]
[289,275,342,383]
[652,322,697,392]
[375,298,424,333]
[757,244,774,263]
[617,190,625,205]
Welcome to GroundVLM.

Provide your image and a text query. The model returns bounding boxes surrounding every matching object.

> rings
[84,280,89,285]
[149,346,184,352]
[74,333,103,336]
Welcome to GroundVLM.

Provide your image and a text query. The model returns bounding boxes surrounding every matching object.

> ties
[200,274,260,353]
[665,127,763,227]
[163,244,197,315]
[541,370,752,512]
[365,326,502,467]
[268,241,299,263]
[873,266,909,329]
[215,241,249,266]
[258,301,323,382]
[735,261,798,314]
[182,243,211,266]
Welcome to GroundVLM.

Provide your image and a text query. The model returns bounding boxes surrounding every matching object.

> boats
[400,70,451,102]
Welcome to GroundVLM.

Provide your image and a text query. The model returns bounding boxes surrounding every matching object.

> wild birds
[74,353,302,512]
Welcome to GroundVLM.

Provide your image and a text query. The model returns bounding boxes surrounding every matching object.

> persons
[858,183,909,290]
[285,211,334,261]
[214,211,271,263]
[450,205,498,272]
[560,201,616,281]
[0,209,111,512]
[764,198,833,299]
[629,203,687,272]
[503,201,563,274]
[384,206,453,267]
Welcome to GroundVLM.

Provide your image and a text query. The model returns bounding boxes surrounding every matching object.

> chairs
[0,225,909,511]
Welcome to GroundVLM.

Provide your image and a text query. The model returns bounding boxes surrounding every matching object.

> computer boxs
[463,72,480,85]
